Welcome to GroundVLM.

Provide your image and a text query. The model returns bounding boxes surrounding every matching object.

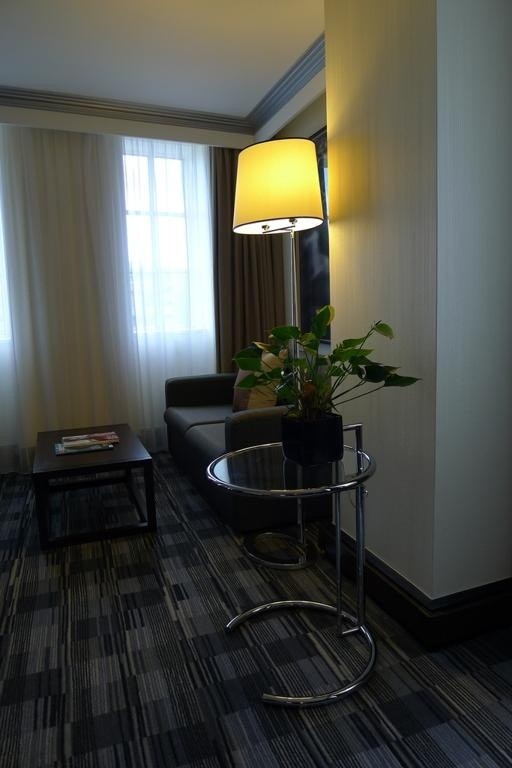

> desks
[206,423,377,707]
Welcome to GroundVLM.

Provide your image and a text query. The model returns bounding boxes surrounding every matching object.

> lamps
[233,138,325,572]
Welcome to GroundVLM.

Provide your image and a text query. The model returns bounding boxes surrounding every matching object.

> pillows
[231,343,288,412]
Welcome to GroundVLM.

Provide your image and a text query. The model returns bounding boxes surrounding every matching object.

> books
[53,443,114,455]
[62,431,120,448]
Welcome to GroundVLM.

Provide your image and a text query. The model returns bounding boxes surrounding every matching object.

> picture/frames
[295,126,332,345]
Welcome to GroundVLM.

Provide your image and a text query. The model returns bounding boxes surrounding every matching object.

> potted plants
[232,306,425,464]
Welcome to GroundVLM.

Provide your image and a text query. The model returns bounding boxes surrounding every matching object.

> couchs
[165,374,331,538]
